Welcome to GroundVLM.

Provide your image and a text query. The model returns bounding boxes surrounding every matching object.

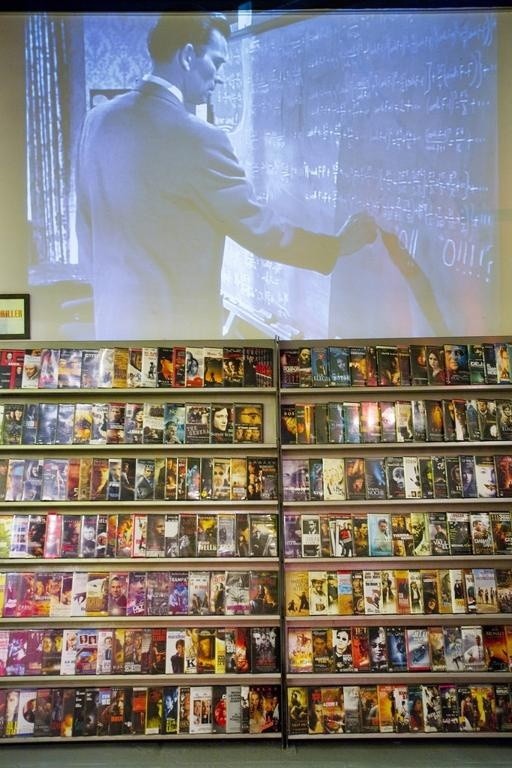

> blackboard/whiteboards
[203,11,510,347]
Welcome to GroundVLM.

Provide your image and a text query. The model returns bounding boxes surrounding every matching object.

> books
[284,568,512,734]
[1,347,278,501]
[278,345,512,558]
[0,513,282,735]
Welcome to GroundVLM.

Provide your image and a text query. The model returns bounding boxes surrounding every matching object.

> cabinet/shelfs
[0,338,512,750]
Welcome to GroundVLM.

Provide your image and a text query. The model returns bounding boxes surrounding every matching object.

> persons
[72,12,378,341]
